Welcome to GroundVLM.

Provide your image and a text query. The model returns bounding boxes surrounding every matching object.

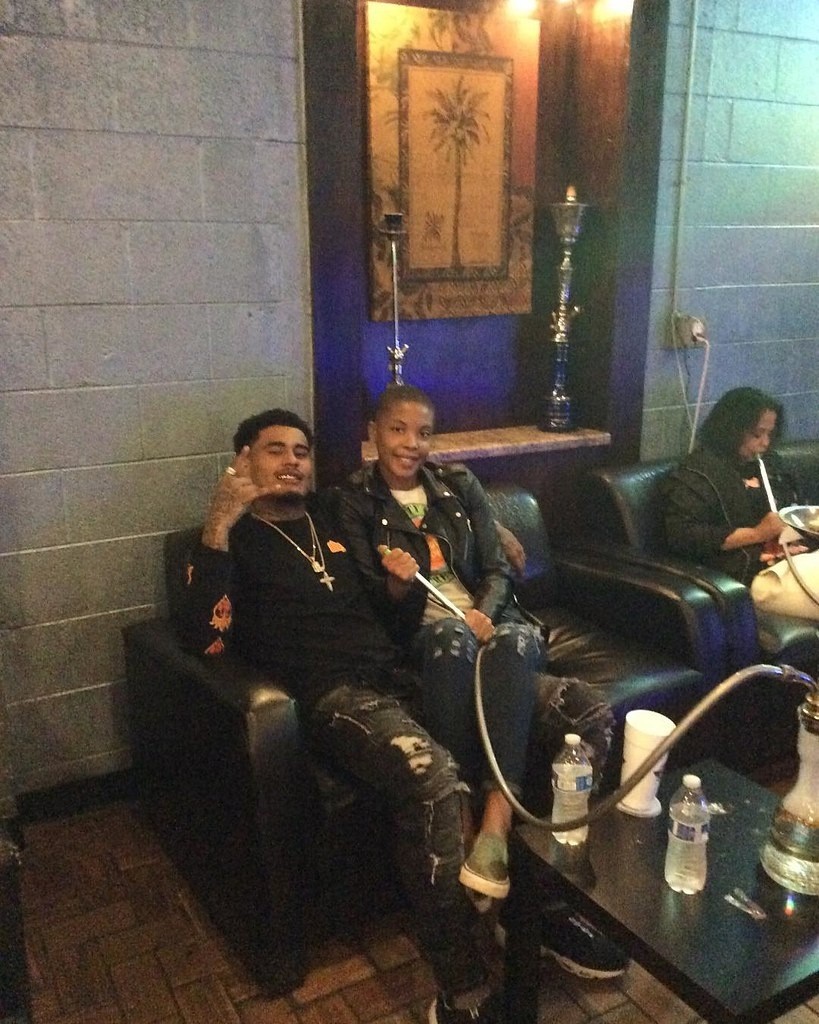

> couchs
[122,479,748,997]
[557,439,819,680]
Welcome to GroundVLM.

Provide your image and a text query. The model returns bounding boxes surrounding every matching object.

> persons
[664,385,819,671]
[184,408,632,1024]
[336,385,551,913]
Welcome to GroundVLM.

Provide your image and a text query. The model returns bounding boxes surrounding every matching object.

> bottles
[551,733,593,847]
[664,774,711,896]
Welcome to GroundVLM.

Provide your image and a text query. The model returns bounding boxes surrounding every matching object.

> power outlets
[662,315,709,351]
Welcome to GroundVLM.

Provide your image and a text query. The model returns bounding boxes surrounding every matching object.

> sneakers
[494,889,626,979]
[460,833,492,913]
[428,995,507,1024]
[458,832,512,898]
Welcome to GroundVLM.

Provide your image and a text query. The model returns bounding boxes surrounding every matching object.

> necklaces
[319,553,335,592]
[250,510,323,573]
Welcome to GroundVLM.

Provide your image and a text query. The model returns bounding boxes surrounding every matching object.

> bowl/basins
[778,505,819,544]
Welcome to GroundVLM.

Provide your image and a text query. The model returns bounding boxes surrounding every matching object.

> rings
[226,466,236,476]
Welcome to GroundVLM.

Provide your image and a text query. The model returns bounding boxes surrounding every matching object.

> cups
[618,709,677,811]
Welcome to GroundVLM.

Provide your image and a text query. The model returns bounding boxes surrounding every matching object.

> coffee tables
[503,757,819,1024]
